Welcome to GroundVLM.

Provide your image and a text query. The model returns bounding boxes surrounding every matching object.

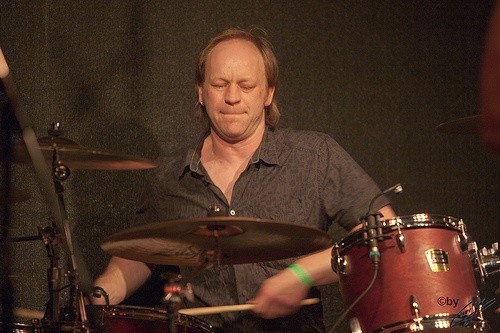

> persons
[85,22,396,333]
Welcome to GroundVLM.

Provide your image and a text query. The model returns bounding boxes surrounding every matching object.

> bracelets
[287,263,313,289]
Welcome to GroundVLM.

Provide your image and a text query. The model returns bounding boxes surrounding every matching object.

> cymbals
[99,211,332,266]
[4,135,158,170]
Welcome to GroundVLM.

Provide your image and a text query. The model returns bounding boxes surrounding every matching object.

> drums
[331,213,484,333]
[13,322,34,333]
[58,304,214,333]
